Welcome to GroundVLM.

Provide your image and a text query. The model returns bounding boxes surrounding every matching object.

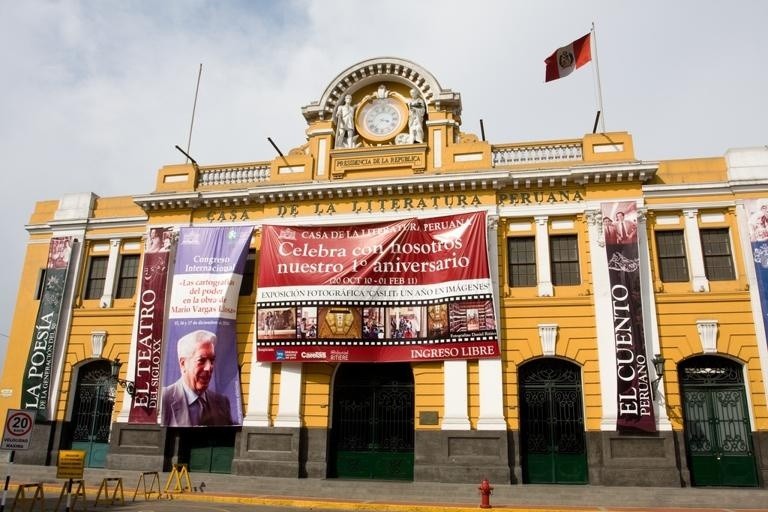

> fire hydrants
[479,478,493,508]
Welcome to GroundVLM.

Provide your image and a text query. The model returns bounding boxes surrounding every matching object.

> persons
[161,330,235,426]
[261,309,316,340]
[389,89,427,146]
[364,309,421,340]
[335,93,373,151]
[49,239,70,268]
[604,212,638,245]
[150,228,164,253]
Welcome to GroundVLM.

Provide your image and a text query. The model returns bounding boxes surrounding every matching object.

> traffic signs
[0,408,37,450]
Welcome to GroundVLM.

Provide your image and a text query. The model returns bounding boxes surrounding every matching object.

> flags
[541,32,592,86]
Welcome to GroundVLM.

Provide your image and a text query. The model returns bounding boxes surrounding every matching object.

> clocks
[363,103,401,137]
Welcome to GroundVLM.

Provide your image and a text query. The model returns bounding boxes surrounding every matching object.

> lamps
[110,359,135,395]
[650,354,667,401]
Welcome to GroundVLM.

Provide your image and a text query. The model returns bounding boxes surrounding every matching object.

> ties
[607,226,610,238]
[621,222,627,241]
[198,398,210,423]
